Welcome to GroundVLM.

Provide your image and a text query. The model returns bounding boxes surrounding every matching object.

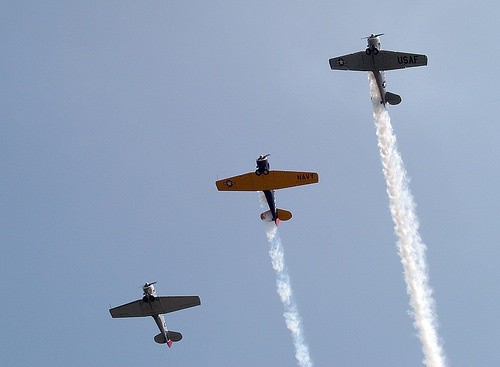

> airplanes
[108,281,201,349]
[215,153,319,226]
[328,32,429,109]
